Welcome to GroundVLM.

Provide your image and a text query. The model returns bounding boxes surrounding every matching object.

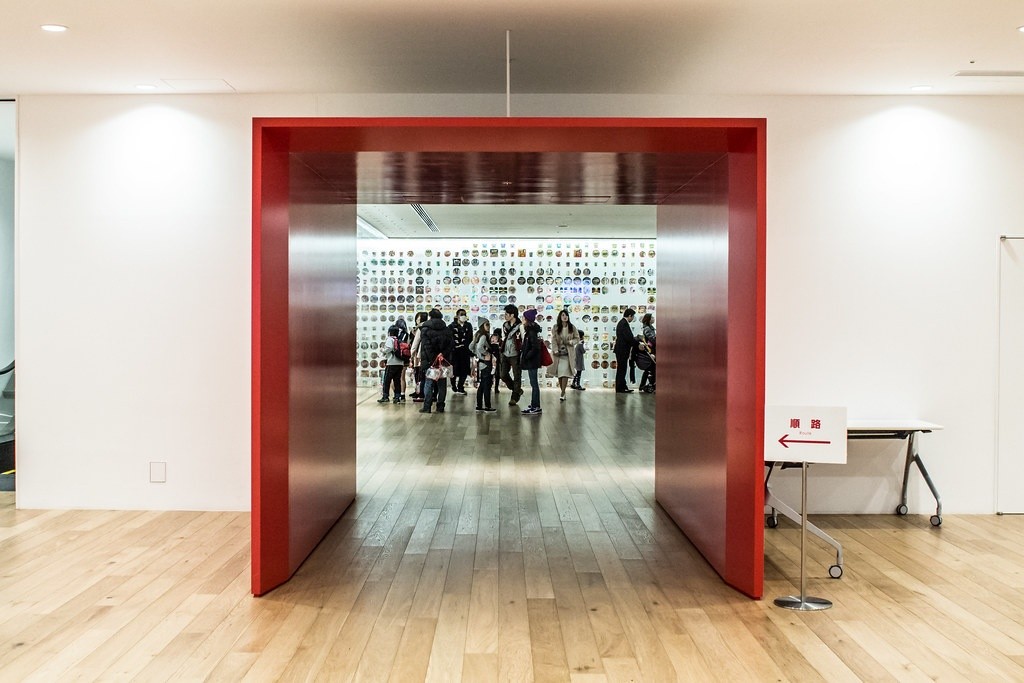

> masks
[458,316,465,322]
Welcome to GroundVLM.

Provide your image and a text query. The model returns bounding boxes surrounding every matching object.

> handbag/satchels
[540,339,552,366]
[405,368,416,388]
[557,345,568,355]
[443,358,453,378]
[425,356,441,380]
[439,356,450,378]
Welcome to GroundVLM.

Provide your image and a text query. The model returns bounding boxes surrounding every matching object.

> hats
[478,315,488,327]
[523,309,537,322]
[578,330,584,337]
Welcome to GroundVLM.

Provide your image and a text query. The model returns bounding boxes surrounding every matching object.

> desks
[764,420,945,526]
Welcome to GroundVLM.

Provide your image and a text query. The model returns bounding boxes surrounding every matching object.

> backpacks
[390,336,411,361]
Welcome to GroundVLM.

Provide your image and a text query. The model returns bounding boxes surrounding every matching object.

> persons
[497,303,523,406]
[570,329,587,391]
[376,308,504,414]
[548,310,580,401]
[638,313,656,393]
[637,334,655,360]
[519,308,545,415]
[612,308,646,392]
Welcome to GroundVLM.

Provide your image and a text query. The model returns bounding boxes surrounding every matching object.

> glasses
[461,313,466,316]
[486,323,490,326]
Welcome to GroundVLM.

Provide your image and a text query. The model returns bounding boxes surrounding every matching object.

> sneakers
[521,408,538,415]
[529,405,543,413]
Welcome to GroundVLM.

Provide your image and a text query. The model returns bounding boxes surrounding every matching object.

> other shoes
[521,390,523,394]
[628,390,635,391]
[452,384,457,392]
[393,399,400,404]
[564,395,567,399]
[509,400,516,406]
[413,398,424,402]
[476,408,484,411]
[409,392,416,396]
[412,394,419,398]
[400,397,406,403]
[378,398,389,402]
[560,393,565,400]
[457,387,467,394]
[436,408,444,411]
[419,408,432,413]
[484,408,497,413]
[617,390,631,393]
